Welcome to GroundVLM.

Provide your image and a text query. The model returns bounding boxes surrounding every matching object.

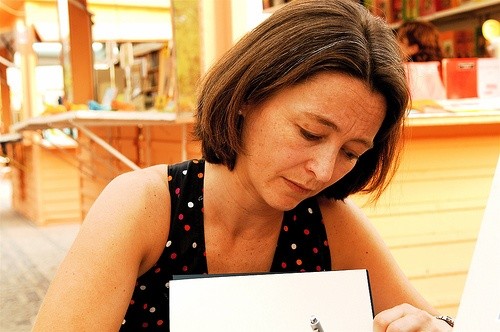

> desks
[0,111,500,332]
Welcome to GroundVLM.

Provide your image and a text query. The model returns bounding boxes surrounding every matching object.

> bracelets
[435,315,454,328]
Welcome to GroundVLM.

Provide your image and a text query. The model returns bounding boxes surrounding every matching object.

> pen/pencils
[310,317,325,331]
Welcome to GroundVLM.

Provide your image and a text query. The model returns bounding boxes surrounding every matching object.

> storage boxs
[403,58,498,105]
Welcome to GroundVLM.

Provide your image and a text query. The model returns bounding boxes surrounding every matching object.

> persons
[397,20,444,62]
[34,0,454,332]
